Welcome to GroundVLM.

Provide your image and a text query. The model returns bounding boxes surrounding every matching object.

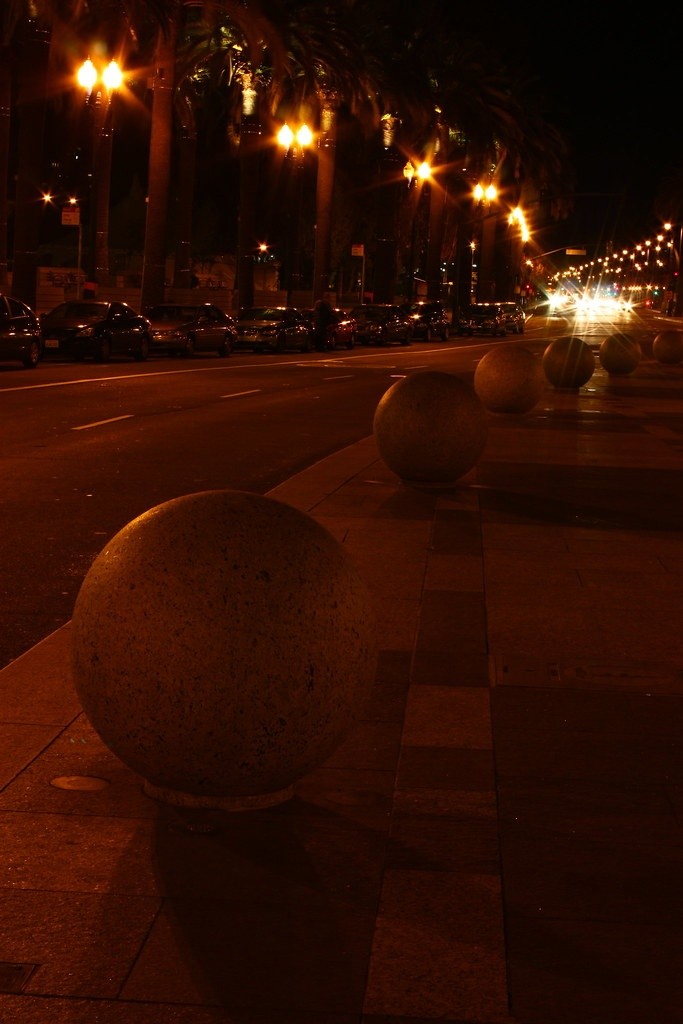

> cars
[41,299,153,363]
[349,303,414,346]
[504,302,526,334]
[0,294,42,369]
[231,306,314,354]
[457,303,507,338]
[400,302,450,340]
[143,303,238,358]
[303,308,357,350]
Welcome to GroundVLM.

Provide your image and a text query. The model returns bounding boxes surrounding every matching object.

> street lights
[72,50,536,303]
[548,220,678,299]
